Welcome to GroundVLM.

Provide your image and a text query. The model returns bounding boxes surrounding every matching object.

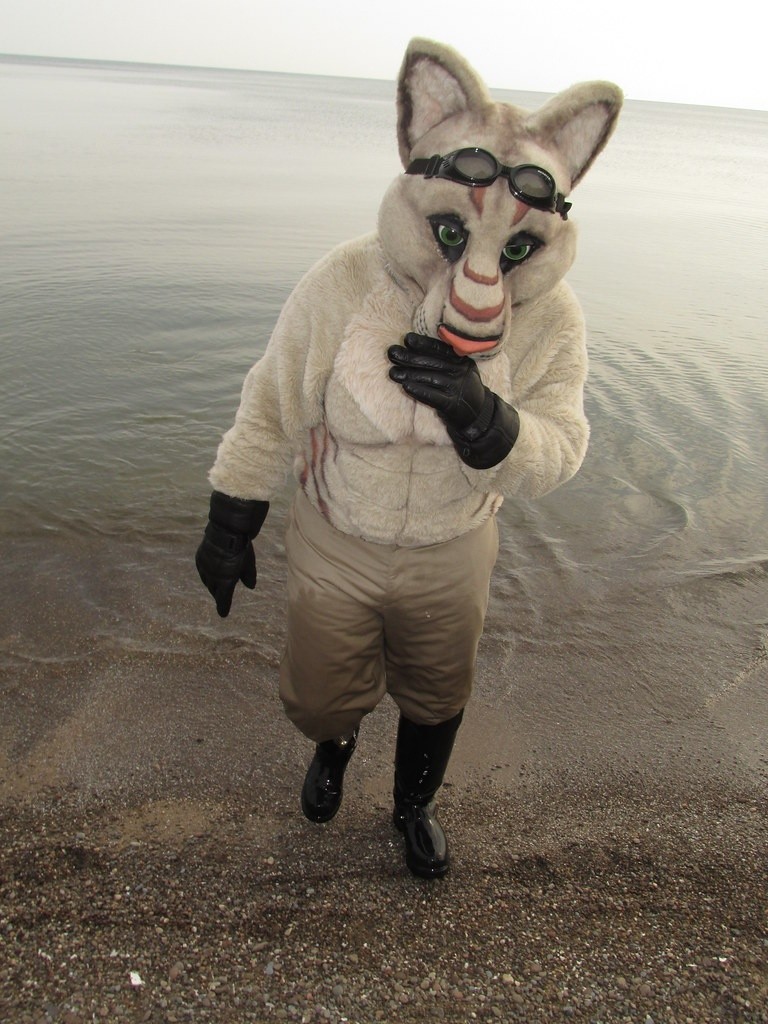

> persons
[196,201,623,877]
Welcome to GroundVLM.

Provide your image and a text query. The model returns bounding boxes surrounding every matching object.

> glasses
[435,147,556,209]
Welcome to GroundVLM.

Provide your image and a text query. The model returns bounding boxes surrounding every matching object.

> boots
[302,736,359,823]
[394,714,464,878]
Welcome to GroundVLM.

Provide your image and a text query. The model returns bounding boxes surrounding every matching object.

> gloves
[196,490,271,617]
[387,332,520,471]
